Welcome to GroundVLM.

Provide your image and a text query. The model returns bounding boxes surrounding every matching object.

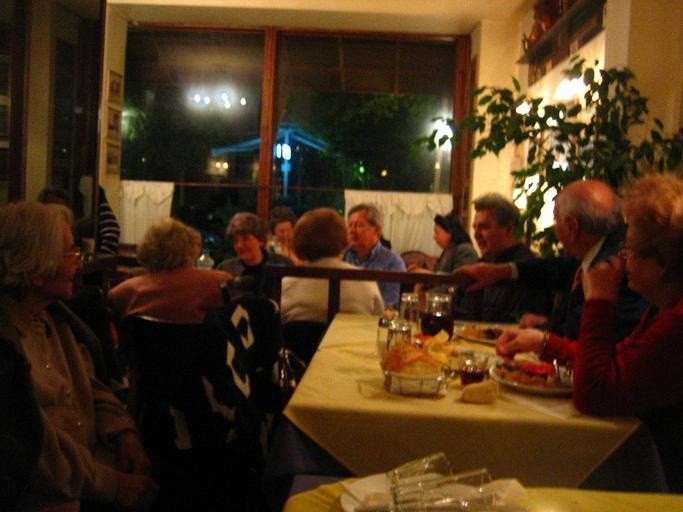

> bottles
[376,314,391,354]
[529,20,543,46]
[421,292,454,339]
[386,321,410,353]
[196,248,215,270]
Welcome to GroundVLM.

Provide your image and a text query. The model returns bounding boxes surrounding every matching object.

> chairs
[66,266,331,498]
[400,251,428,269]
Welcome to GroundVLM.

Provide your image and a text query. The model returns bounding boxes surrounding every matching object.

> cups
[543,14,560,32]
[388,450,499,511]
[81,233,96,257]
[455,355,489,386]
[401,293,419,333]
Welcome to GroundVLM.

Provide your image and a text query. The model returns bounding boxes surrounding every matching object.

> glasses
[50,245,83,264]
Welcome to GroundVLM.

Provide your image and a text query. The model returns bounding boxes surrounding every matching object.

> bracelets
[541,328,548,356]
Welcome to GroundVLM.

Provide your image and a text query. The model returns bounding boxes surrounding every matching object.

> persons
[452,193,533,322]
[496,172,683,492]
[1,188,404,511]
[452,180,649,340]
[407,215,479,319]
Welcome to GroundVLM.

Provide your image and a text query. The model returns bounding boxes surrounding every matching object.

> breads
[379,345,442,392]
[461,380,499,403]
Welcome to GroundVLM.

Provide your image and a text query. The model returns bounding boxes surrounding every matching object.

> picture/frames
[105,68,123,110]
[99,142,120,176]
[100,103,121,146]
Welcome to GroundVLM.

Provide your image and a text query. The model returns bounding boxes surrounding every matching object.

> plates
[443,349,497,373]
[454,323,500,344]
[488,362,573,397]
[336,474,393,512]
